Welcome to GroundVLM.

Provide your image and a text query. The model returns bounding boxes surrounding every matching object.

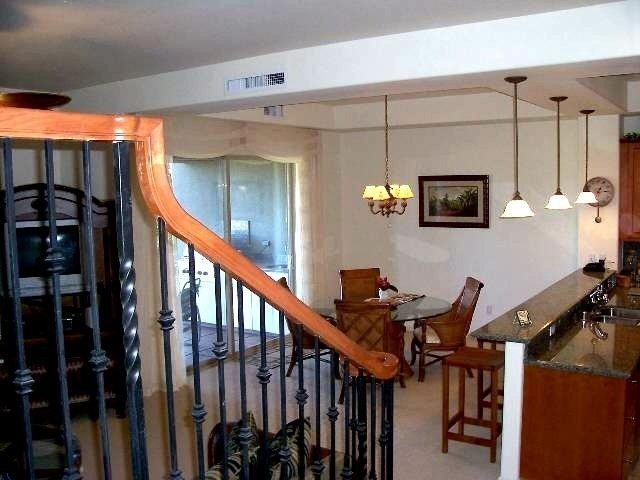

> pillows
[202,412,313,480]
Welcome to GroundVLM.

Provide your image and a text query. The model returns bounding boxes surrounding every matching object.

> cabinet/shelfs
[619,141,640,242]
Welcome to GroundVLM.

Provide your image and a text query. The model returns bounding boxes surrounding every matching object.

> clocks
[583,178,614,223]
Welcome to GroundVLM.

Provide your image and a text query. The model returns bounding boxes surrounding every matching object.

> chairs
[276,268,484,402]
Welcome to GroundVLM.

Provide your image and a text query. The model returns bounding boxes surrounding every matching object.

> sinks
[591,315,639,325]
[600,306,640,319]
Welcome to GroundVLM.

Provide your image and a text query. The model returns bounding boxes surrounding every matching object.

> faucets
[590,284,608,307]
[590,323,609,340]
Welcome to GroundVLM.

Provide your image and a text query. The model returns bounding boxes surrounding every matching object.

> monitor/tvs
[220,220,250,247]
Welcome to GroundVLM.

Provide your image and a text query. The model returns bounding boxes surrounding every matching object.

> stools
[442,337,505,463]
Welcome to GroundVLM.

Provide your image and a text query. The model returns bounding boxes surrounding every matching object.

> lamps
[361,76,599,218]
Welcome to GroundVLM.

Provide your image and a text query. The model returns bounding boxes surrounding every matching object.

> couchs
[208,420,350,480]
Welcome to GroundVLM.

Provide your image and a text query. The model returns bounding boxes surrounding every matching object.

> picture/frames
[418,174,490,228]
